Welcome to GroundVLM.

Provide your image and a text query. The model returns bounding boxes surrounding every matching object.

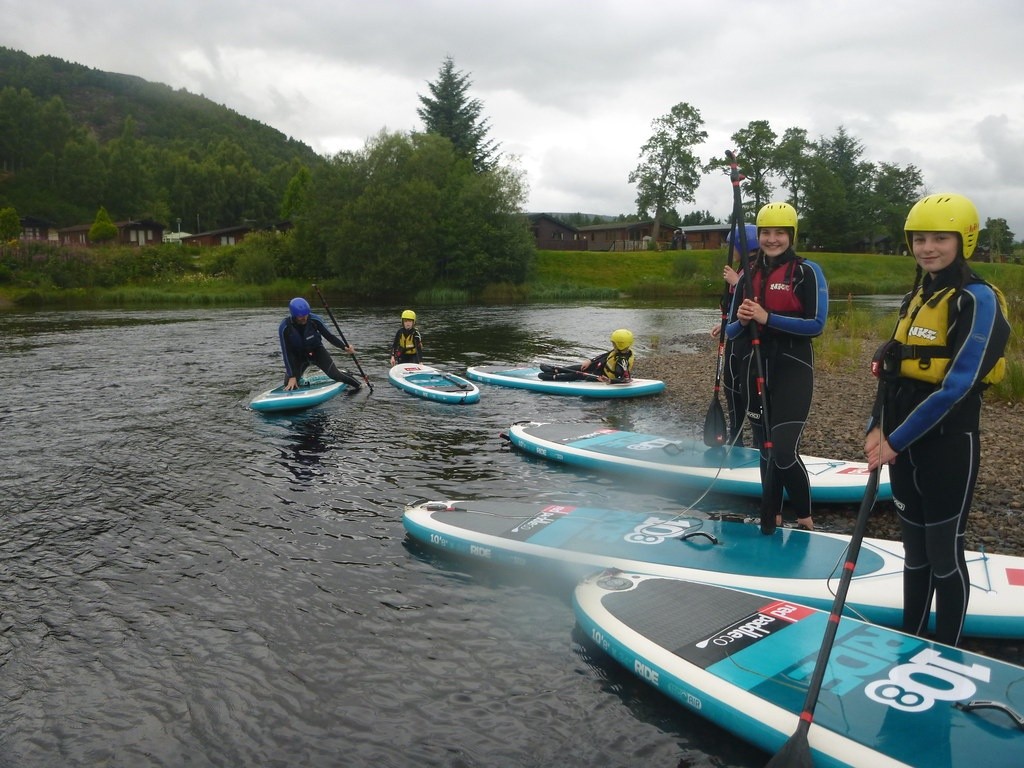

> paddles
[726,149,786,534]
[704,175,745,448]
[317,290,374,390]
[761,464,883,768]
[553,364,633,385]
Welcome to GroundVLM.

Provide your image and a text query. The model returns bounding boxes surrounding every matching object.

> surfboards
[572,571,1024,768]
[250,370,347,411]
[389,363,480,404]
[509,421,892,500]
[403,498,1024,637]
[466,367,664,398]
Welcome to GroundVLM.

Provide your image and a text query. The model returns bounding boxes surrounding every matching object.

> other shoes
[538,373,555,381]
[540,363,556,373]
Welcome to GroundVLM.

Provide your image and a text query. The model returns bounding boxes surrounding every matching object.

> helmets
[401,310,416,326]
[288,298,310,320]
[727,226,759,257]
[903,195,981,260]
[611,329,633,350]
[755,203,798,246]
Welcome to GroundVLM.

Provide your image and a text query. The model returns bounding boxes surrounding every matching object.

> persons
[862,191,1013,649]
[390,309,425,367]
[279,298,363,391]
[723,202,825,532]
[710,225,767,459]
[539,329,636,384]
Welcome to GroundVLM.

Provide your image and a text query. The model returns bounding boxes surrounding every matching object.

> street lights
[177,218,181,241]
